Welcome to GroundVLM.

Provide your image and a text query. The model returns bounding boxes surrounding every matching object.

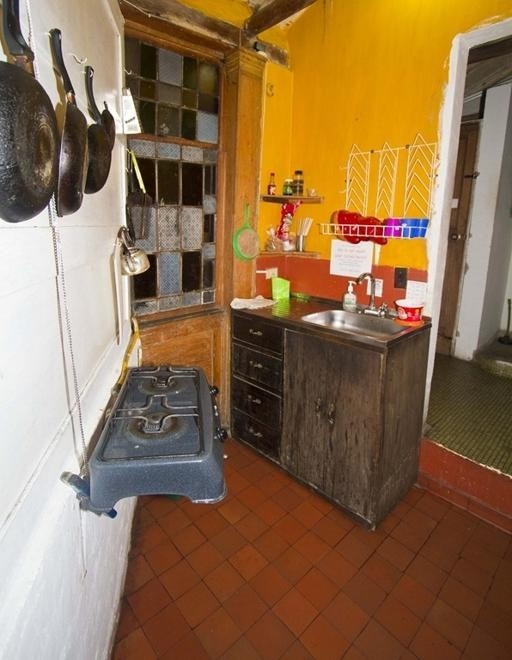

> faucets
[358,272,373,311]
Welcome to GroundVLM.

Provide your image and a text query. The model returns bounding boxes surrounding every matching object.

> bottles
[267,170,302,197]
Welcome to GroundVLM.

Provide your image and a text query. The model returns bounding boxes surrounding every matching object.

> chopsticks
[296,218,313,236]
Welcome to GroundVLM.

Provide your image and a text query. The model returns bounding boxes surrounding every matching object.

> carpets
[424,355,511,479]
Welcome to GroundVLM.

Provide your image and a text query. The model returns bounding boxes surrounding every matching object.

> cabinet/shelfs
[257,195,322,258]
[226,309,282,463]
[281,328,430,534]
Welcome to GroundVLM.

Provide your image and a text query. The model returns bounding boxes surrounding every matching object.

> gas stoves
[89,363,227,513]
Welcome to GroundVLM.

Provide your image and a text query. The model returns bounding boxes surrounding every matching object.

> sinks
[301,308,412,336]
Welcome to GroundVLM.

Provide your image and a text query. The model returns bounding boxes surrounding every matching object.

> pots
[234,203,262,262]
[1,0,115,222]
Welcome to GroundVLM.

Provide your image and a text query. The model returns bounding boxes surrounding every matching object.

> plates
[331,211,386,244]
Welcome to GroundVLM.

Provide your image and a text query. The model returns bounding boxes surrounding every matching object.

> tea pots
[118,228,150,278]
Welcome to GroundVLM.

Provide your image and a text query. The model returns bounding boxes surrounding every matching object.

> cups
[306,188,318,197]
[294,235,305,253]
[396,299,423,321]
[383,216,428,238]
